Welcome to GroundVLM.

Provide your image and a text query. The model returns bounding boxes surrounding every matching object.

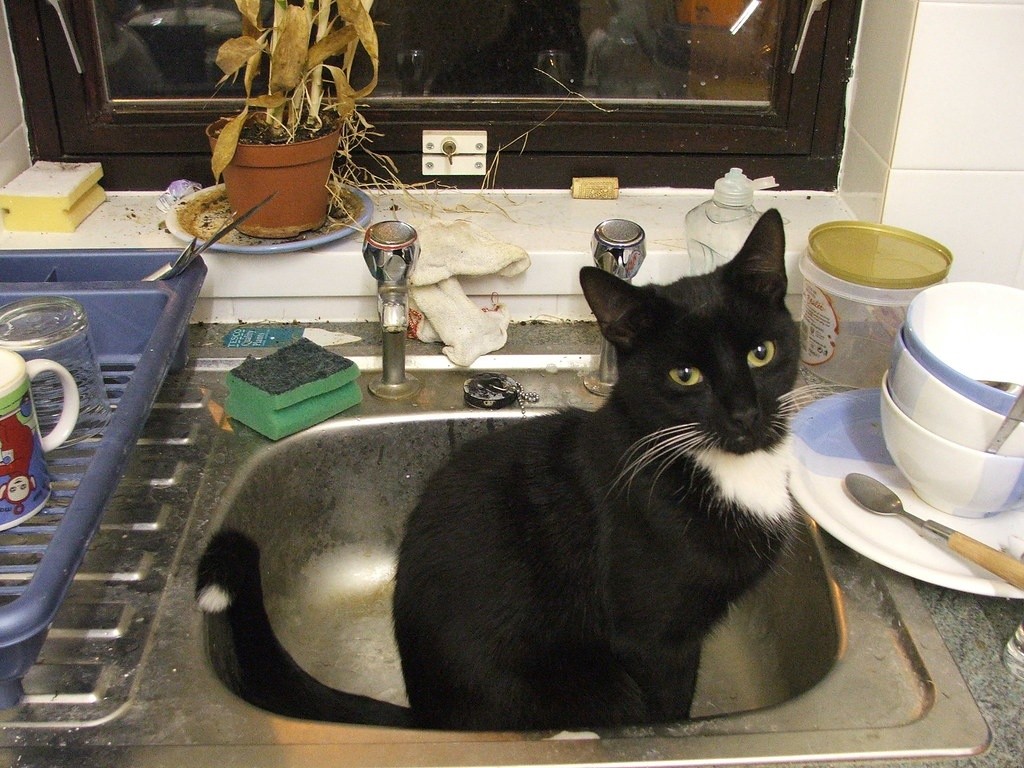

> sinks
[124,368,994,768]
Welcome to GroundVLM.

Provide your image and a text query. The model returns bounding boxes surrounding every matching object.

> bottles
[684,167,766,276]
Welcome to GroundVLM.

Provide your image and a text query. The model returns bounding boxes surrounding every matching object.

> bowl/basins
[880,283,1023,520]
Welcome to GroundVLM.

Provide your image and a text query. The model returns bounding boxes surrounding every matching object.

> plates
[782,387,1024,600]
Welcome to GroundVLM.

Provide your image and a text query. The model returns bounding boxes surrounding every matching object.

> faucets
[359,218,426,403]
[583,218,650,399]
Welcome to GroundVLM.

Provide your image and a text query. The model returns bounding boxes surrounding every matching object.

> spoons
[845,472,1024,591]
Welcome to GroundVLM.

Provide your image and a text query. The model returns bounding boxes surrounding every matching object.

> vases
[206,114,342,237]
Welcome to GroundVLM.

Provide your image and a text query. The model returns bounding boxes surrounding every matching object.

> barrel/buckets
[797,221,953,390]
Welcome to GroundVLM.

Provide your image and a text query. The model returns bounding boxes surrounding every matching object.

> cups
[0,347,80,534]
[0,295,112,450]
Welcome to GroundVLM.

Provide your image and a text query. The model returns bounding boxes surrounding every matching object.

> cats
[192,207,804,735]
